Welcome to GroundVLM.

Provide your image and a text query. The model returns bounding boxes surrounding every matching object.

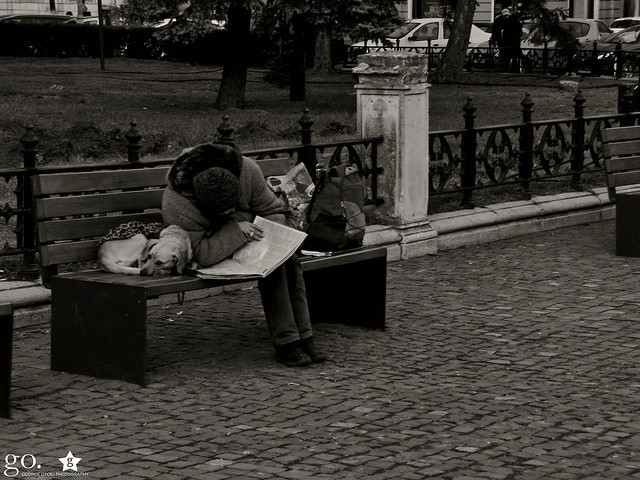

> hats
[192,167,241,211]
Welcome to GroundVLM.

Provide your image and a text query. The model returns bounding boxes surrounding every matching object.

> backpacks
[303,163,366,252]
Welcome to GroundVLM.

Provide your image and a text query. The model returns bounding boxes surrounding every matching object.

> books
[194,214,309,281]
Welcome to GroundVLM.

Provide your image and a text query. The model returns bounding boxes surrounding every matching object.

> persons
[160,141,327,368]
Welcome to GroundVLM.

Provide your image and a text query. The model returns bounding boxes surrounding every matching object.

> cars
[348,18,493,62]
[520,18,617,63]
[471,19,551,72]
[579,24,640,59]
[608,16,640,43]
[72,17,136,25]
[153,18,227,30]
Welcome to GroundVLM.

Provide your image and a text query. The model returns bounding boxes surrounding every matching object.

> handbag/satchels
[267,162,315,218]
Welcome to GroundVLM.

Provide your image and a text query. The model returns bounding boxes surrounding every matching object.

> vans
[0,13,76,25]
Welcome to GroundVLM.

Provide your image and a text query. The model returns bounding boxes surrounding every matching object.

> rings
[249,232,254,238]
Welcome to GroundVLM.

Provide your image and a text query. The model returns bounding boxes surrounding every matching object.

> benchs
[597,125,639,257]
[25,157,385,387]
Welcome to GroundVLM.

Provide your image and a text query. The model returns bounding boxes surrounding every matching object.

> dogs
[97,224,194,277]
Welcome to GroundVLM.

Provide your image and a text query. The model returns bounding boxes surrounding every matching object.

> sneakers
[300,336,326,363]
[275,341,312,366]
